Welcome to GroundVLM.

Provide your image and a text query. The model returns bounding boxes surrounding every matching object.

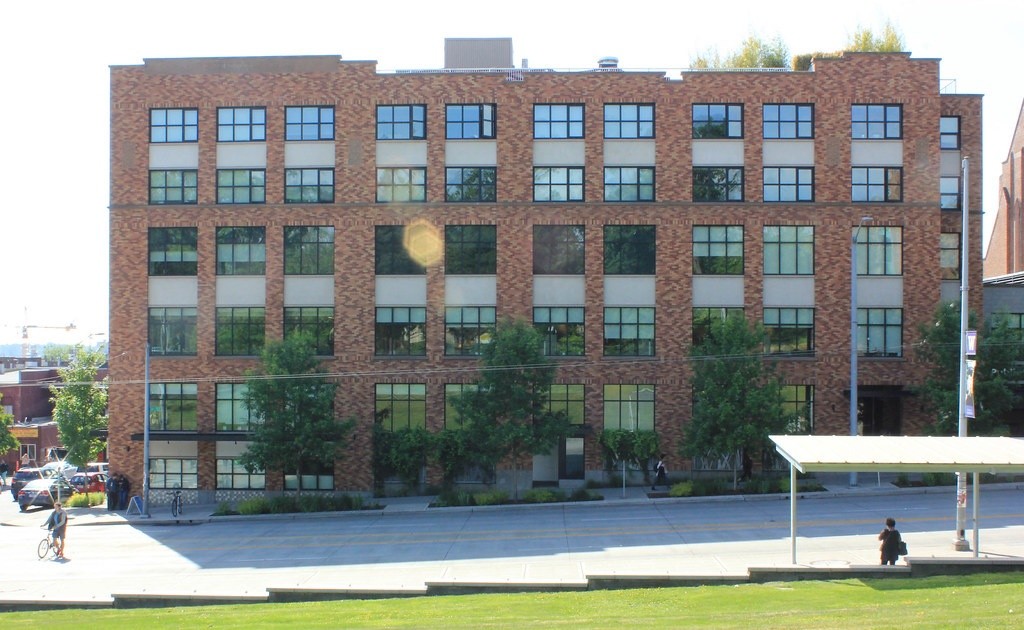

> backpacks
[654,462,663,473]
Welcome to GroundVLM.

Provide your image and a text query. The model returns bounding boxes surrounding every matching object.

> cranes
[2,305,76,360]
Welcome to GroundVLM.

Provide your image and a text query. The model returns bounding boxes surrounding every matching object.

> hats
[660,453,666,460]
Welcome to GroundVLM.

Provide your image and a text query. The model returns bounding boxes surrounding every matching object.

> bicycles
[37,527,62,559]
[165,489,183,517]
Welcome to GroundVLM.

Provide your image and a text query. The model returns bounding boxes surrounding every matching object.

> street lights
[138,342,151,518]
[848,216,877,487]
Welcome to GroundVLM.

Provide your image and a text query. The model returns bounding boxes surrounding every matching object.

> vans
[76,462,109,474]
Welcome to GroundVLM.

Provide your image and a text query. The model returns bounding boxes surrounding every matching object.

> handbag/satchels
[880,543,885,551]
[898,542,907,555]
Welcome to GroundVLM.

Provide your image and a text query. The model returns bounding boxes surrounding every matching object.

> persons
[879,517,901,566]
[105,472,129,511]
[20,454,29,467]
[0,459,9,494]
[736,448,753,485]
[40,502,67,558]
[652,454,671,490]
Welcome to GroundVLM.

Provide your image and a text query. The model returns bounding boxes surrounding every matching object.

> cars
[43,461,78,480]
[70,472,111,494]
[17,479,76,512]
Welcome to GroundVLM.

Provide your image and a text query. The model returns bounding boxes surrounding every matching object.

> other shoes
[57,549,63,558]
[651,487,657,491]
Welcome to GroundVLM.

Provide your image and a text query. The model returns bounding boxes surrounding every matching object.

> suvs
[11,467,62,502]
